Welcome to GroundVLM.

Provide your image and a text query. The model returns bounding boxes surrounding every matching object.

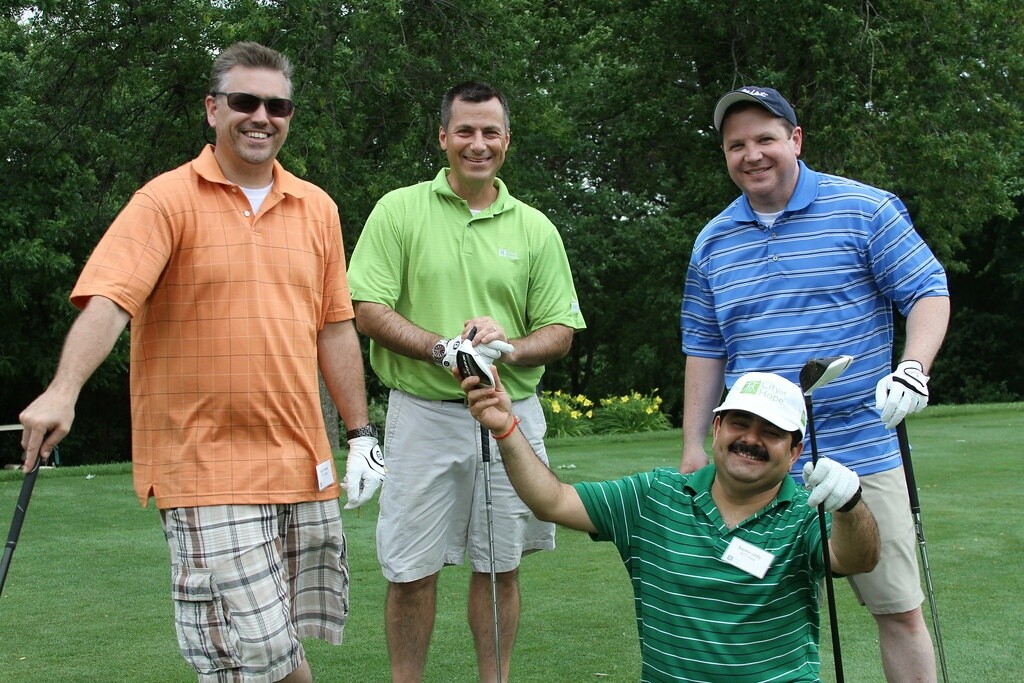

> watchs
[346,423,379,439]
[432,336,450,367]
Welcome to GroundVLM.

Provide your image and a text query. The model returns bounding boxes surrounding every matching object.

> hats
[713,371,808,443]
[713,85,801,128]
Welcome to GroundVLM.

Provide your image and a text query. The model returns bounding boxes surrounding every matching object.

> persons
[18,42,385,683]
[680,85,952,682]
[345,83,576,683]
[451,362,882,683]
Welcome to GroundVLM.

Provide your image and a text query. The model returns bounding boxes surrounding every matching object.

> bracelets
[483,416,518,439]
[837,487,863,511]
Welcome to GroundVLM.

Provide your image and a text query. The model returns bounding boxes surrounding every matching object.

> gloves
[874,360,930,429]
[441,326,514,390]
[340,436,387,509]
[802,458,863,514]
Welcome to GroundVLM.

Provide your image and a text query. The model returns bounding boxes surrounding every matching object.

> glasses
[209,91,295,117]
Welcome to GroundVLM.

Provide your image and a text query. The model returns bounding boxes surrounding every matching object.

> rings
[494,326,497,332]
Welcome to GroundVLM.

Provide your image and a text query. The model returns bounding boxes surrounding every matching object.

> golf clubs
[0,431,51,596]
[478,423,502,683]
[887,384,950,683]
[798,353,856,683]
